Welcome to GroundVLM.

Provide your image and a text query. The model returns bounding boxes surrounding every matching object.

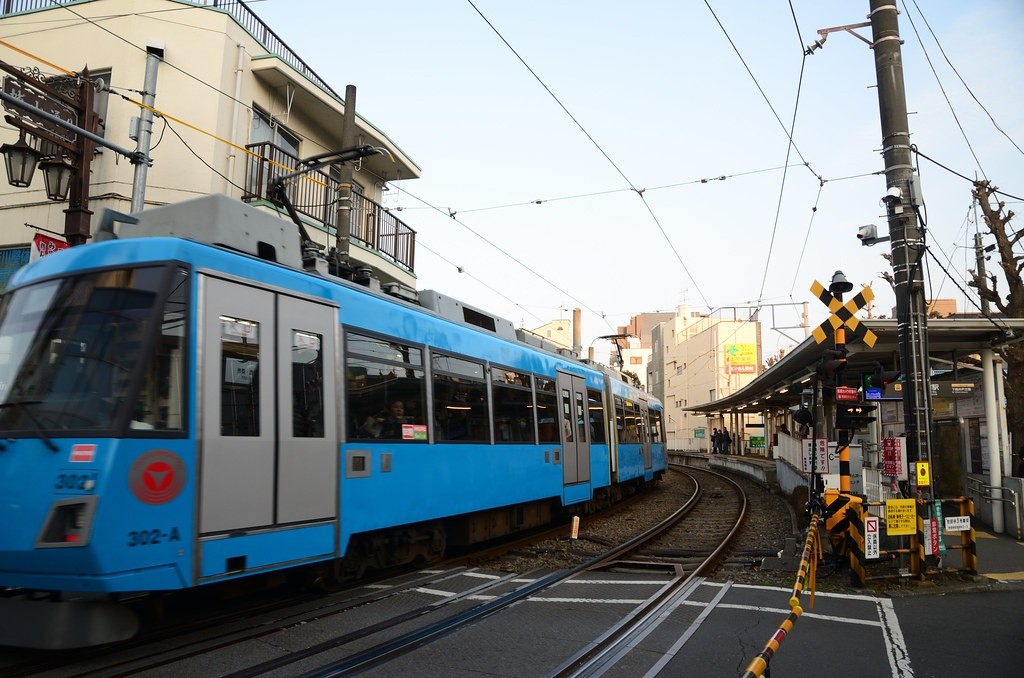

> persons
[34,344,91,397]
[565,419,572,439]
[374,400,406,439]
[780,424,790,435]
[710,427,729,454]
[794,424,809,438]
[622,423,639,443]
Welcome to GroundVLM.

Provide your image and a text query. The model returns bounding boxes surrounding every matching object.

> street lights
[0,60,99,247]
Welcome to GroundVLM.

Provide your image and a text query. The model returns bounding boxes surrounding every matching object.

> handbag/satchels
[726,437,732,444]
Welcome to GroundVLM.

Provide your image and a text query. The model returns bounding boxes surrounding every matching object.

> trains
[0,236,668,593]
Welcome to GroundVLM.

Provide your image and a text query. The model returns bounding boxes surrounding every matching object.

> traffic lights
[871,370,901,388]
[820,349,847,381]
[835,401,877,430]
